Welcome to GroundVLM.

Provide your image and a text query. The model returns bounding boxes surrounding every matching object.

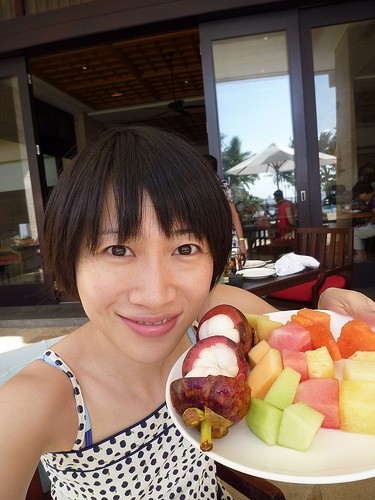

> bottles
[231,230,241,273]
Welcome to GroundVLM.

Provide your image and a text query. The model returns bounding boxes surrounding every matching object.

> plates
[166,308,375,484]
[244,260,265,269]
[236,267,275,280]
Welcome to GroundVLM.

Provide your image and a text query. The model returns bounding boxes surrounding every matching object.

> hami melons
[244,313,282,399]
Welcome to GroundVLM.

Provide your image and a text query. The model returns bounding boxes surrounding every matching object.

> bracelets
[239,237,245,241]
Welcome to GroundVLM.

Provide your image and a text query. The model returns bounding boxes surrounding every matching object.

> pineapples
[303,346,337,378]
[338,351,375,435]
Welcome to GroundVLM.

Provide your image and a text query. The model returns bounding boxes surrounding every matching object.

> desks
[242,268,316,297]
[323,210,370,221]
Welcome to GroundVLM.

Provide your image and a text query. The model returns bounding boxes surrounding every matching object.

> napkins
[274,251,320,277]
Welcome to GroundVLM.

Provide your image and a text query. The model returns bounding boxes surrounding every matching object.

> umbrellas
[223,143,337,191]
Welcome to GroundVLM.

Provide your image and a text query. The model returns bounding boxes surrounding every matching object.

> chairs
[0,242,41,284]
[262,226,355,311]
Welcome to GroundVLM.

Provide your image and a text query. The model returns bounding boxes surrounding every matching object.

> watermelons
[294,378,341,430]
[282,349,308,382]
[267,323,313,351]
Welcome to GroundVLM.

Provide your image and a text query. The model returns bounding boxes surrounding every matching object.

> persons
[234,181,375,263]
[201,155,248,261]
[0,126,375,500]
